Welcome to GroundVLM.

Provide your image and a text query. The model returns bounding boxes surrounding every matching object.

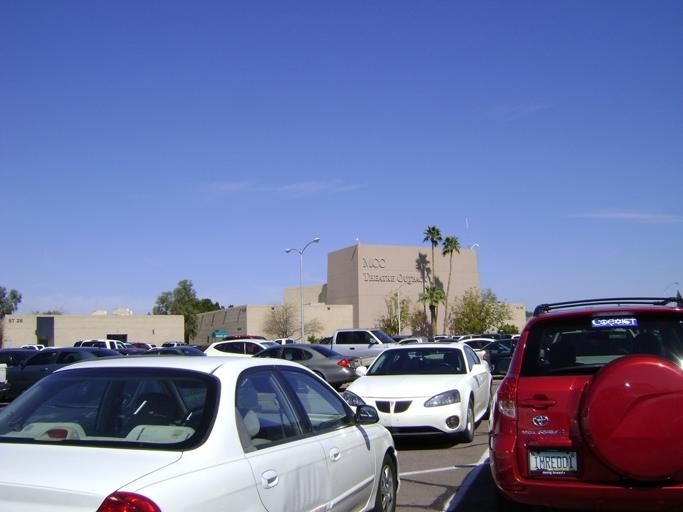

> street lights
[284,238,322,345]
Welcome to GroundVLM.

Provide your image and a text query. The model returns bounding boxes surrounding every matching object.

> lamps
[130,394,176,425]
[631,332,660,355]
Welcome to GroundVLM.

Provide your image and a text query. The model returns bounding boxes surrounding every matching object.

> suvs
[203,338,291,360]
[488,292,683,509]
[222,334,266,340]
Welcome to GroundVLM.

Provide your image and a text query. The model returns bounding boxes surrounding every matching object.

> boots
[488,292,683,509]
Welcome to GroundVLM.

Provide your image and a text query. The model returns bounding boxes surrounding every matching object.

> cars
[2,329,208,409]
[1,354,401,512]
[472,337,520,377]
[341,340,494,444]
[392,331,520,366]
[251,343,363,389]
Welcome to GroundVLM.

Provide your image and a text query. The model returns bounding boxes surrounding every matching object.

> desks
[284,238,322,345]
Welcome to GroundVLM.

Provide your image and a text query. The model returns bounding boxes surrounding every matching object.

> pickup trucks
[313,329,398,360]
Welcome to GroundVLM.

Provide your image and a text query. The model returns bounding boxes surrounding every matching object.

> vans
[274,338,294,346]
